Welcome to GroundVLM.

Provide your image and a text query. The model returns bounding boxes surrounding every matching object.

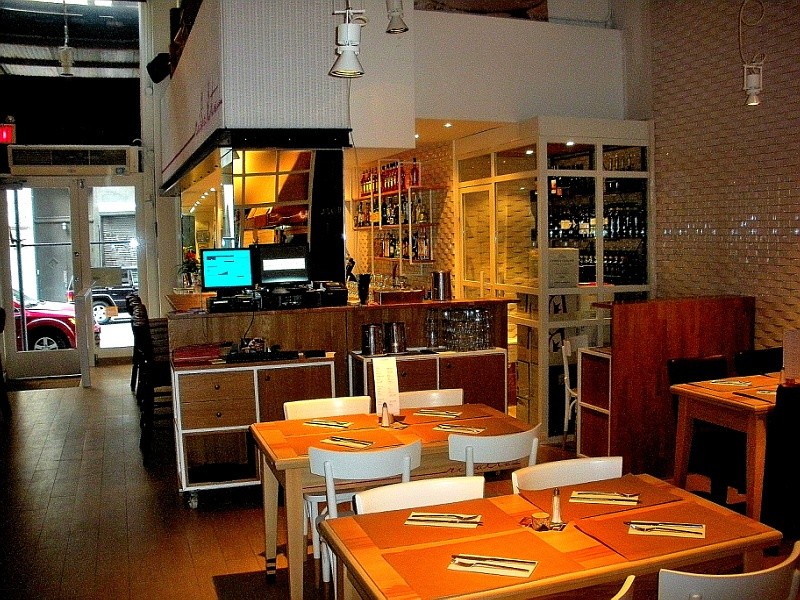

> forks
[630,525,702,535]
[312,420,348,425]
[577,492,640,497]
[451,559,529,572]
[412,514,478,519]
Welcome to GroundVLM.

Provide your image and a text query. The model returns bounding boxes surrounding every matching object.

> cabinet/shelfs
[347,348,509,418]
[353,157,449,278]
[576,347,614,460]
[171,359,337,489]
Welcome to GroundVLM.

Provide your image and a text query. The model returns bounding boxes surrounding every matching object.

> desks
[670,370,800,519]
[249,402,529,599]
[318,470,779,599]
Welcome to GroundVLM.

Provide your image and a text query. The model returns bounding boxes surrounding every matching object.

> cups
[346,273,393,302]
[426,309,495,352]
[361,324,383,356]
[383,322,406,353]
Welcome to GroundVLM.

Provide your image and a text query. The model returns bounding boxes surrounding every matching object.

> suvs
[67,265,138,326]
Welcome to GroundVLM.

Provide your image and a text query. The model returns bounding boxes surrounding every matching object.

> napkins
[413,408,463,419]
[434,422,487,435]
[404,509,481,528]
[322,436,373,449]
[447,553,540,578]
[569,489,639,506]
[304,417,354,430]
[627,519,705,539]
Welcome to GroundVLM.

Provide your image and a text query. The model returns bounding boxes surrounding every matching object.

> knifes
[570,497,640,503]
[406,519,483,524]
[305,421,348,429]
[623,521,702,527]
[451,554,536,565]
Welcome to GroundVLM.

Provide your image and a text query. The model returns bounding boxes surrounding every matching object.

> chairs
[284,334,800,600]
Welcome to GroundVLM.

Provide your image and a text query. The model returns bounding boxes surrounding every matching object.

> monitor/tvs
[200,243,311,300]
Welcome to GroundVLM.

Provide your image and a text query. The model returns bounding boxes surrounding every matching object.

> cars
[12,288,100,351]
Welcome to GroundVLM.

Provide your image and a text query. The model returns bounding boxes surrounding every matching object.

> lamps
[330,0,368,78]
[743,62,764,106]
[386,0,409,34]
[57,0,74,76]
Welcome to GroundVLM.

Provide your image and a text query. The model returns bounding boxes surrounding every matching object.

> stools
[126,293,173,453]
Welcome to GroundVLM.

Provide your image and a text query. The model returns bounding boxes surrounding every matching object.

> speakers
[145,52,171,84]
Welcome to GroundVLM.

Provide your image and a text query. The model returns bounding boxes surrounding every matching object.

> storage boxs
[513,246,614,435]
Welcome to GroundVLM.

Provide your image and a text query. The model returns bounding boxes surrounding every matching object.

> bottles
[549,164,584,196]
[360,161,406,196]
[579,250,647,285]
[549,202,648,242]
[409,158,419,186]
[402,228,430,260]
[605,156,638,194]
[353,195,428,227]
[551,487,563,523]
[381,402,390,426]
[374,229,400,258]
